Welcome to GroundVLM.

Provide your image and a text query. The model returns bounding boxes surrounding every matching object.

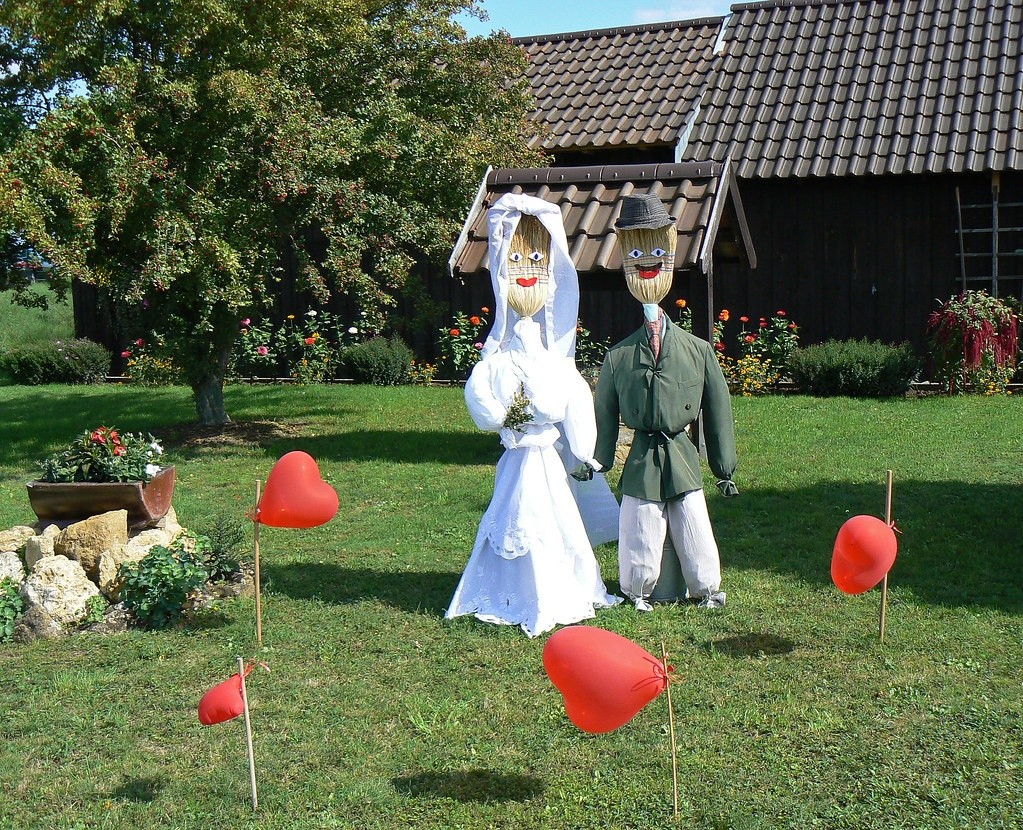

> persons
[437,190,618,638]
[580,192,739,610]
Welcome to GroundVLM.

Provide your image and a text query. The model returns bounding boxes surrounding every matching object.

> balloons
[542,625,673,733]
[251,450,340,529]
[197,674,243,726]
[830,515,898,594]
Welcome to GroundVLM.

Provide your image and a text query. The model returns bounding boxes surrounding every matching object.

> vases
[26,464,176,531]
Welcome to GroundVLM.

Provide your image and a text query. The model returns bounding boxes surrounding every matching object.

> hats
[614,194,677,231]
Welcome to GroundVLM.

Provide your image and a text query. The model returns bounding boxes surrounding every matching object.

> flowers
[34,420,164,486]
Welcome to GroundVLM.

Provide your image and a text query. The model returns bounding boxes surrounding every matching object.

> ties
[643,316,663,367]
